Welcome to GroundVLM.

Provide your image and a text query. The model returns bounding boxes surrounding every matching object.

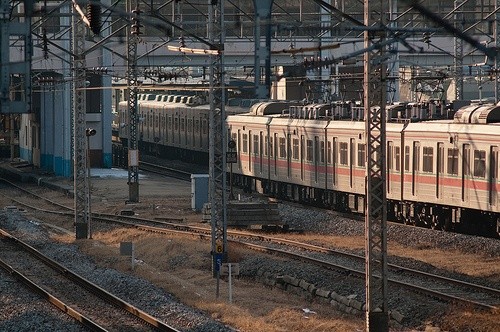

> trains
[117,92,499,240]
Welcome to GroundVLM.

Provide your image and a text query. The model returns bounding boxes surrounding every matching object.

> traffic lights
[86,129,96,137]
